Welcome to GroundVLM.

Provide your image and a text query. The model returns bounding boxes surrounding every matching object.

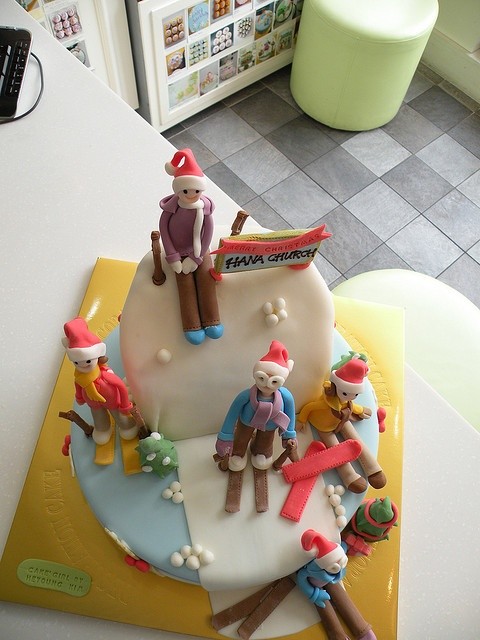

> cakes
[1,149,407,640]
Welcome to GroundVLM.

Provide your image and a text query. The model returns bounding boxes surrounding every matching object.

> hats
[253,340,294,381]
[164,147,207,195]
[330,359,370,394]
[63,316,106,362]
[300,528,345,570]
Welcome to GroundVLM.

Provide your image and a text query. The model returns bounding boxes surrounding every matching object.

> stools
[328,268,477,429]
[289,0,439,132]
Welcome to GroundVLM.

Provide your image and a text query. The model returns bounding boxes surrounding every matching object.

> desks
[0,0,478,636]
